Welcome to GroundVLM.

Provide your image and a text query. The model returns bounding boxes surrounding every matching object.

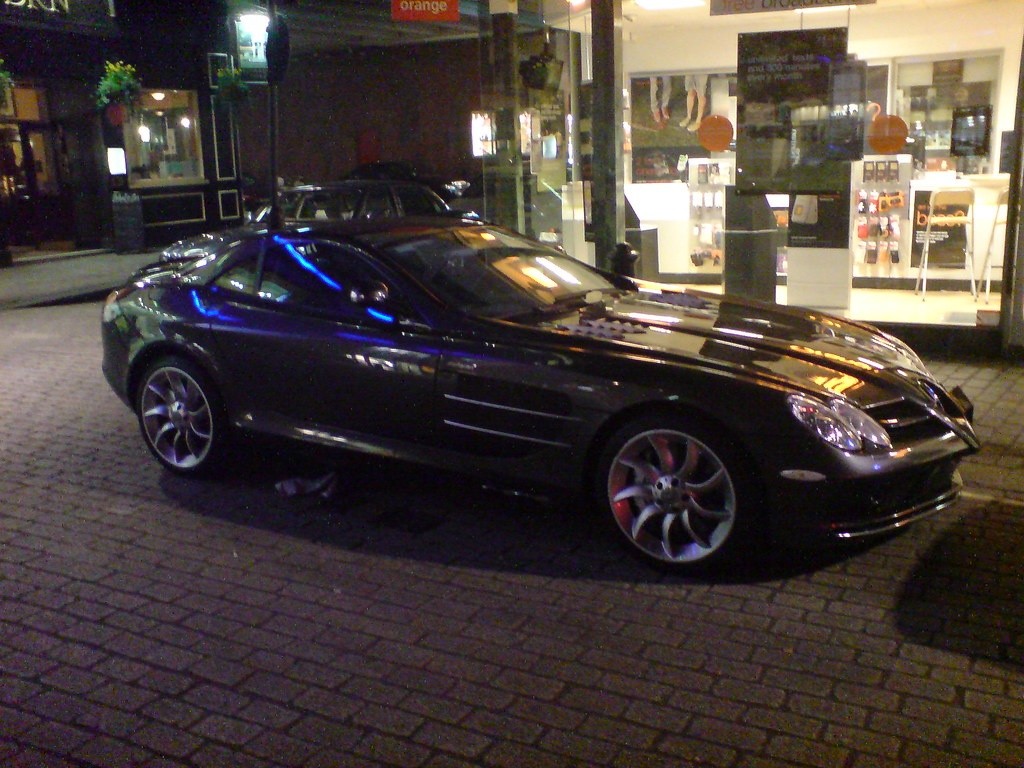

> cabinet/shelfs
[687,157,734,248]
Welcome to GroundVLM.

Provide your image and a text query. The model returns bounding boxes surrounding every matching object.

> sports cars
[98,216,982,580]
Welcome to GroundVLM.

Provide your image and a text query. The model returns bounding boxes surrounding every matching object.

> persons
[679,73,709,132]
[649,74,671,122]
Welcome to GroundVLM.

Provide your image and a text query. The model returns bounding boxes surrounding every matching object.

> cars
[245,178,454,225]
[351,157,426,184]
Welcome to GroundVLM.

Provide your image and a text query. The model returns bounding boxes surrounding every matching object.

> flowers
[214,67,243,86]
[94,60,143,112]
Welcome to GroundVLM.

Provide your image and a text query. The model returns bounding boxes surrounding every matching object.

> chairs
[912,186,1009,304]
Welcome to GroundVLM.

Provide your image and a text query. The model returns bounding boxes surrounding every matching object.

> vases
[104,101,124,126]
[213,82,245,100]
